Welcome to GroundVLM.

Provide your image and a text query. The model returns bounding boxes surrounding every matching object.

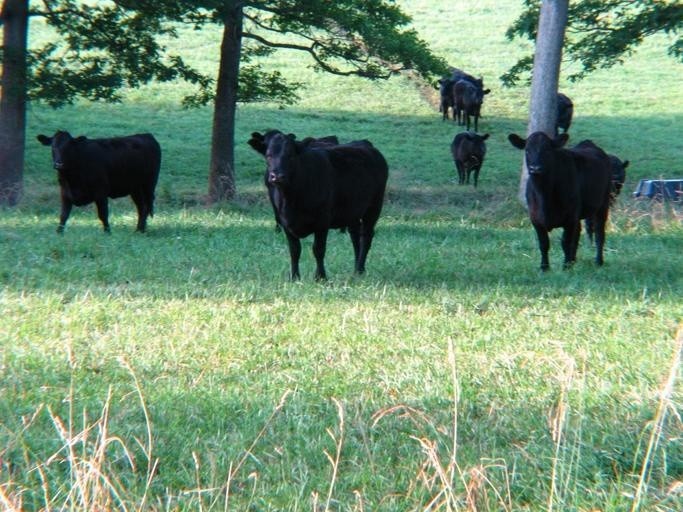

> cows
[608,155,630,208]
[36,129,162,234]
[437,66,491,133]
[259,133,389,281]
[555,93,573,136]
[449,132,490,189]
[508,130,613,271]
[247,128,346,233]
